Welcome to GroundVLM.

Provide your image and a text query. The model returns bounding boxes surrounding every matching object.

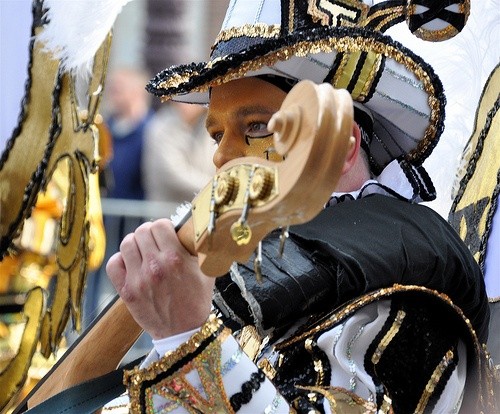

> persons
[21,0,492,414]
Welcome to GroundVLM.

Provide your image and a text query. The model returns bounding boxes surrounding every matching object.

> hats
[144,0,472,203]
